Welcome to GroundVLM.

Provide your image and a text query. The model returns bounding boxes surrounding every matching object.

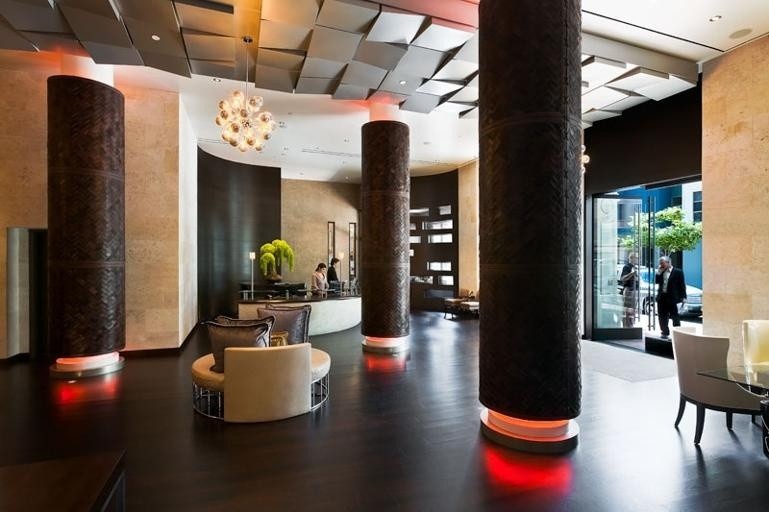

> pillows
[204,303,312,375]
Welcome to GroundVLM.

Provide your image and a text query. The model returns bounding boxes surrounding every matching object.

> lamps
[216,37,276,154]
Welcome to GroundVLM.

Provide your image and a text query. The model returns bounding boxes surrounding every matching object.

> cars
[636,266,702,316]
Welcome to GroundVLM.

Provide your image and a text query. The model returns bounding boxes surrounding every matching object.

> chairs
[671,318,768,445]
[444,288,480,320]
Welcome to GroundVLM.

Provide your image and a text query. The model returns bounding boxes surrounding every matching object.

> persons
[656,257,686,335]
[311,263,329,295]
[620,253,639,328]
[327,258,339,284]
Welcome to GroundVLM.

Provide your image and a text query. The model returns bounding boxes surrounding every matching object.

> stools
[191,346,331,423]
